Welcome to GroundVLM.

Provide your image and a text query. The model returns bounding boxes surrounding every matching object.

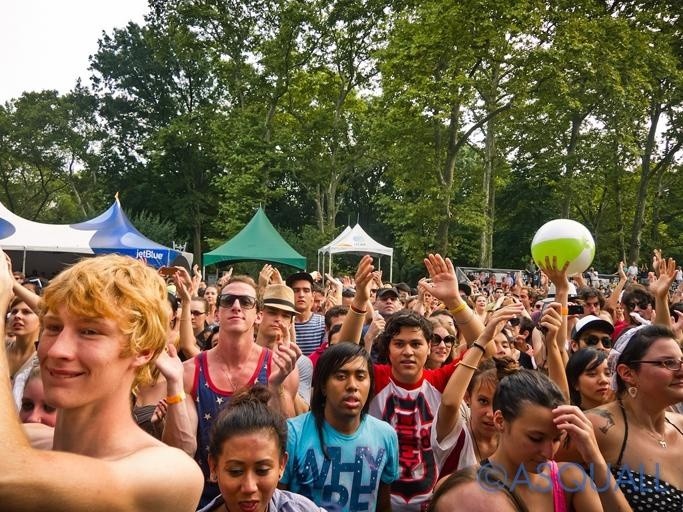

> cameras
[162,267,178,274]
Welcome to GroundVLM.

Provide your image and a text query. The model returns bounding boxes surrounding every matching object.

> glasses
[30,277,42,288]
[217,294,257,310]
[431,333,455,348]
[626,300,651,311]
[342,287,356,294]
[629,358,683,371]
[191,310,205,317]
[579,334,612,349]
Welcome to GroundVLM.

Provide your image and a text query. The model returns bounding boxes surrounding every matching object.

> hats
[570,314,615,341]
[261,284,302,315]
[376,286,400,300]
[539,281,581,304]
[286,272,314,287]
[458,283,472,297]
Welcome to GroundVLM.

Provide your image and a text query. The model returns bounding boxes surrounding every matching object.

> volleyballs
[531,219,596,276]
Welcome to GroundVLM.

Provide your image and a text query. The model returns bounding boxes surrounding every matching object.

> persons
[0,250,204,512]
[423,464,529,512]
[552,325,683,512]
[429,298,570,490]
[432,355,637,511]
[153,275,302,512]
[336,251,498,512]
[191,386,327,511]
[272,342,400,511]
[0,245,683,442]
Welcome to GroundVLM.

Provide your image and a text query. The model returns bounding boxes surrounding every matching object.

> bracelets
[348,305,367,316]
[164,392,185,405]
[456,360,478,371]
[473,342,485,355]
[447,302,467,312]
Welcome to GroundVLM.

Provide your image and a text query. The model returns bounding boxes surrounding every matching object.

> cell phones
[537,302,556,333]
[568,305,584,315]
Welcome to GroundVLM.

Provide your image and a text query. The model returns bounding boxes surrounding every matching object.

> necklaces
[632,415,670,449]
